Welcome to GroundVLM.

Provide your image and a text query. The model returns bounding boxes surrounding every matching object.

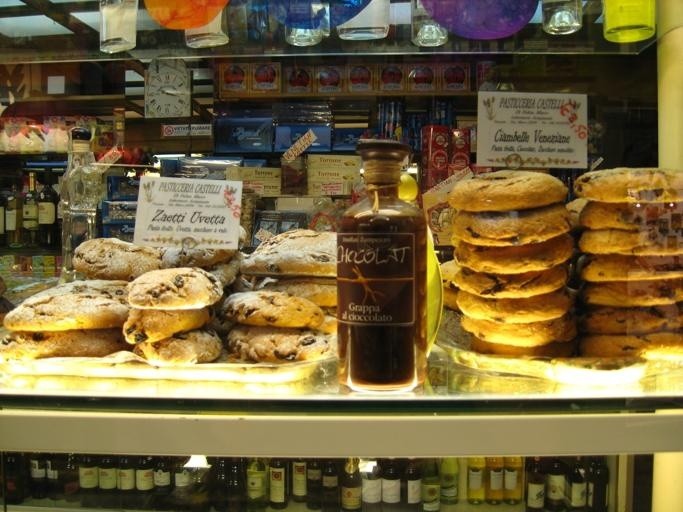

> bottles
[418,459,438,509]
[380,461,400,507]
[466,456,484,505]
[487,457,503,506]
[76,455,99,508]
[28,455,45,502]
[213,458,226,511]
[134,457,152,505]
[586,456,609,511]
[37,171,61,250]
[305,460,321,510]
[175,464,191,505]
[504,456,522,506]
[400,460,421,508]
[289,458,307,503]
[339,458,362,511]
[360,460,380,510]
[566,458,585,510]
[3,455,24,505]
[525,457,545,510]
[267,459,287,508]
[60,126,103,284]
[21,171,38,248]
[321,459,339,510]
[338,139,427,395]
[1,189,8,250]
[225,459,244,511]
[247,459,265,510]
[5,173,26,248]
[440,458,459,505]
[153,459,172,504]
[116,455,135,510]
[545,456,565,509]
[60,454,78,504]
[45,457,61,501]
[98,457,115,509]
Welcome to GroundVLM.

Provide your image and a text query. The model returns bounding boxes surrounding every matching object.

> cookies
[439,168,582,360]
[574,168,682,358]
[5,230,340,362]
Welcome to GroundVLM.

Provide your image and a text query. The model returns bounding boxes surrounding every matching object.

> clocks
[144,68,193,118]
